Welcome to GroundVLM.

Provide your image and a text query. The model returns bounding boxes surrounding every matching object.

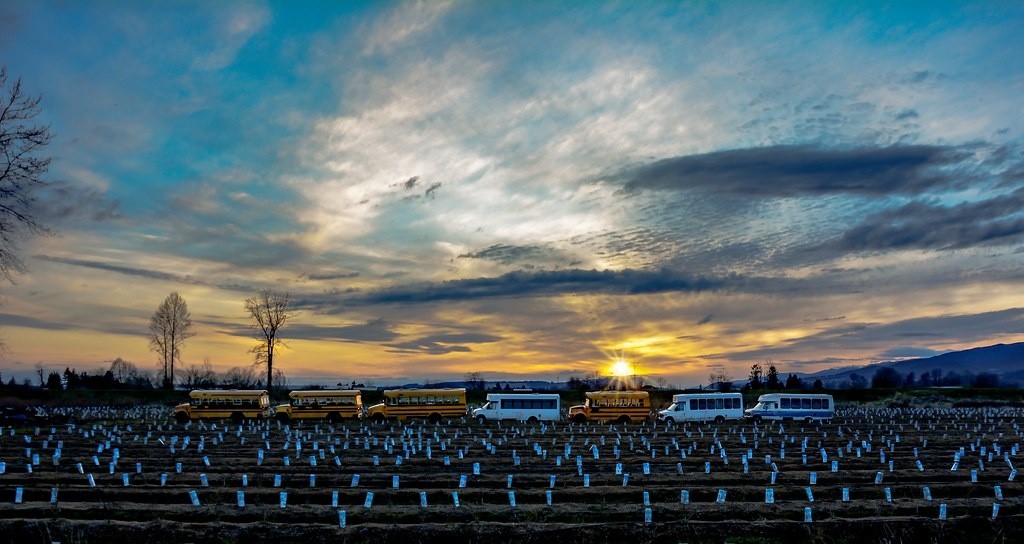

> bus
[275,391,364,424]
[175,390,270,424]
[367,388,468,425]
[473,394,561,425]
[659,393,743,424]
[744,393,834,423]
[569,391,650,426]
